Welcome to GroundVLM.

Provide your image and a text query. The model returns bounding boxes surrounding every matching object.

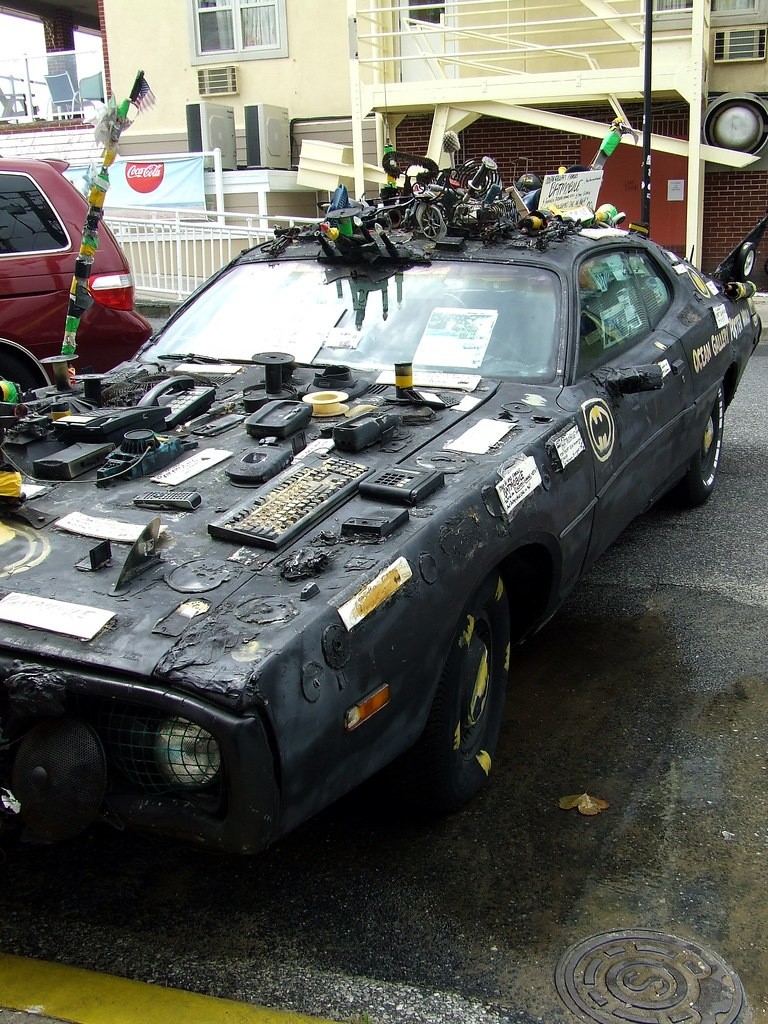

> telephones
[138,376,217,430]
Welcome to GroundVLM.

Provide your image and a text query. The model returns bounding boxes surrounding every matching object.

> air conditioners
[185,102,238,171]
[244,104,291,171]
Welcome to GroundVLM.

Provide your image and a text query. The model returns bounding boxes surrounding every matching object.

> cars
[1,225,763,862]
[1,156,153,412]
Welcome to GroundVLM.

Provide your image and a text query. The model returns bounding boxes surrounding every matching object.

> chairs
[43,71,103,120]
[0,87,45,124]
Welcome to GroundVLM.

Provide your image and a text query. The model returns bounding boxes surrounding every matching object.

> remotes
[135,491,202,511]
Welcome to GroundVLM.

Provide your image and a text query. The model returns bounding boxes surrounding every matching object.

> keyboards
[206,452,376,548]
